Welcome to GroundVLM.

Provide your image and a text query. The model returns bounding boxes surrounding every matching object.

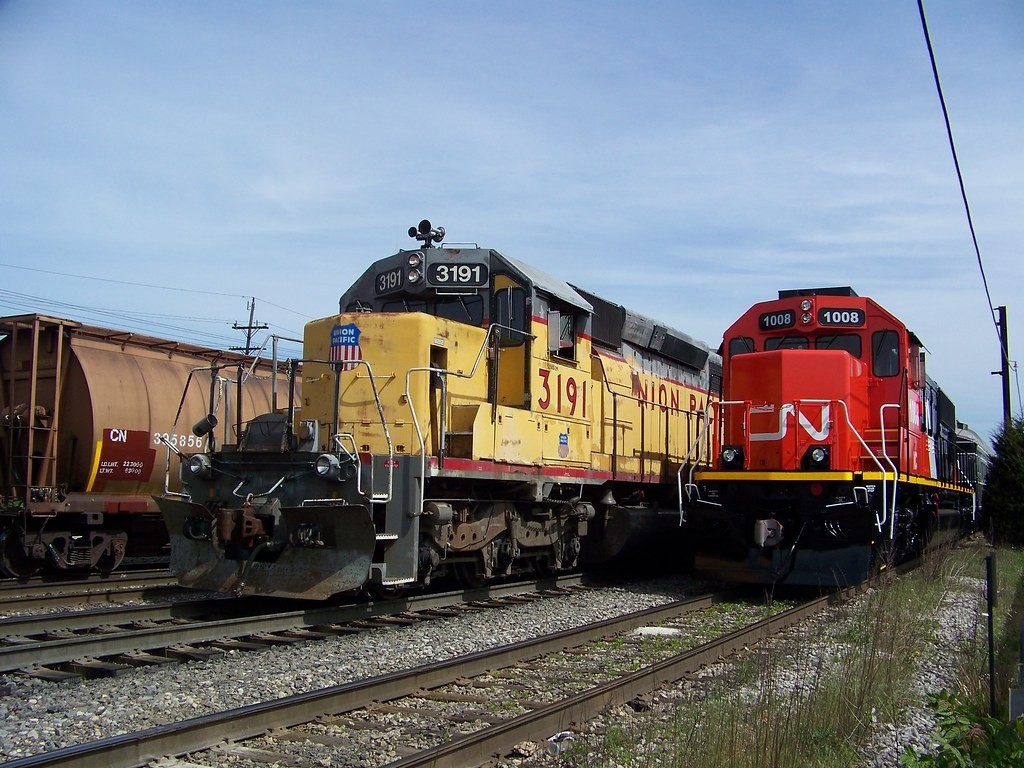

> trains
[1,310,302,582]
[159,218,726,601]
[681,285,993,588]
